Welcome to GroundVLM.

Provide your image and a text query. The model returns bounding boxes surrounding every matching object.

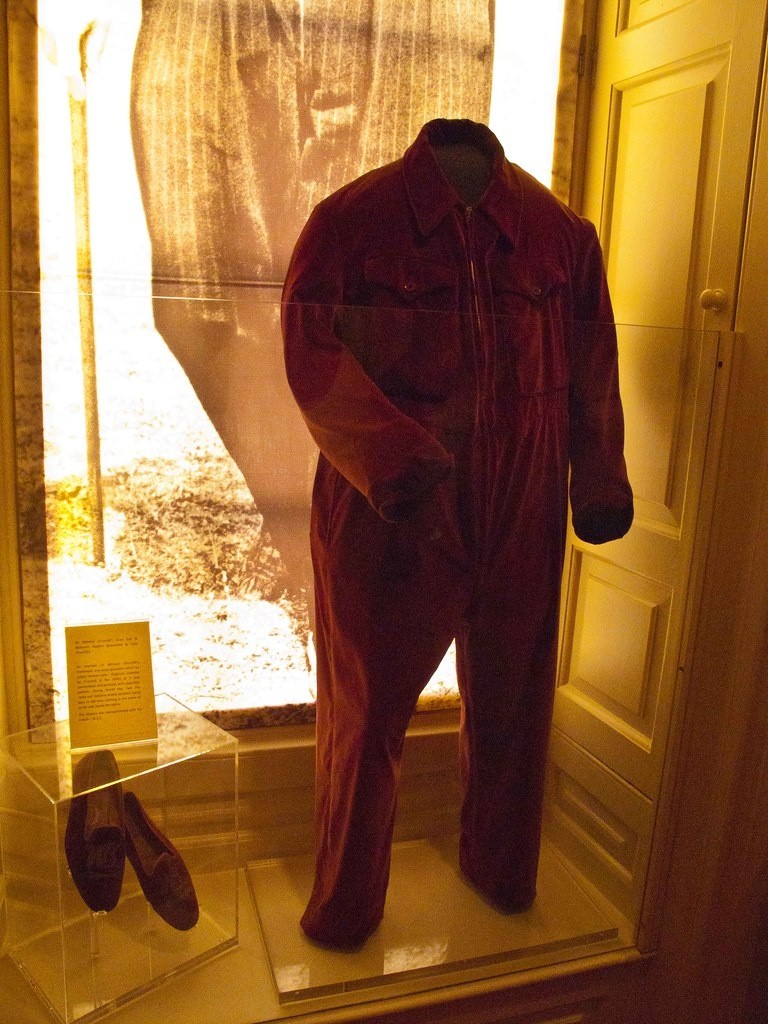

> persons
[280,118,635,952]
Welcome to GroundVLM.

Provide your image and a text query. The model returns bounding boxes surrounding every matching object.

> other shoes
[120,790,199,932]
[66,750,125,911]
